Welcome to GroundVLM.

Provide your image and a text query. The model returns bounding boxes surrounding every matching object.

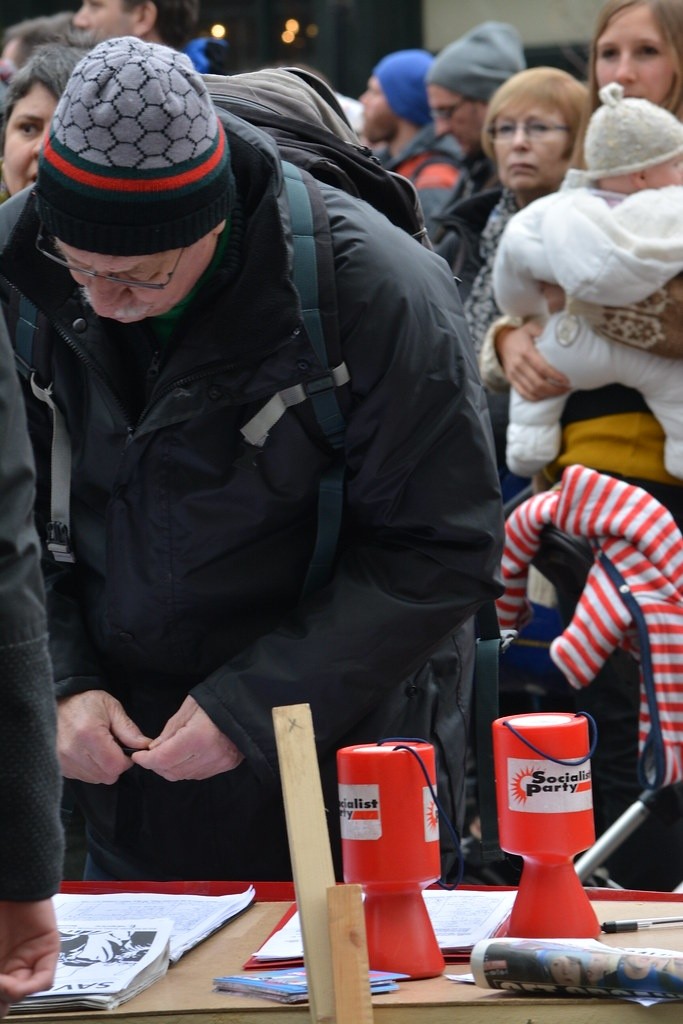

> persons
[0,316,68,1008]
[1,0,682,532]
[1,36,508,880]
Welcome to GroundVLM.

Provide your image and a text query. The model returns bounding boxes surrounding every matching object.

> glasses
[434,97,468,120]
[486,119,571,144]
[34,220,185,289]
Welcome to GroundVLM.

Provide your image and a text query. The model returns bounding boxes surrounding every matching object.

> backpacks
[11,66,430,457]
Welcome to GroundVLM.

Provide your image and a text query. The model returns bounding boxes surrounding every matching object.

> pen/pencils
[600,915,682,934]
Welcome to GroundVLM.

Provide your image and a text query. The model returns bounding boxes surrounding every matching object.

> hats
[584,83,683,178]
[424,21,526,102]
[38,35,233,256]
[373,48,434,126]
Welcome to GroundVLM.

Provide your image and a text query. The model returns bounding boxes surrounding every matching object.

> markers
[573,789,661,886]
